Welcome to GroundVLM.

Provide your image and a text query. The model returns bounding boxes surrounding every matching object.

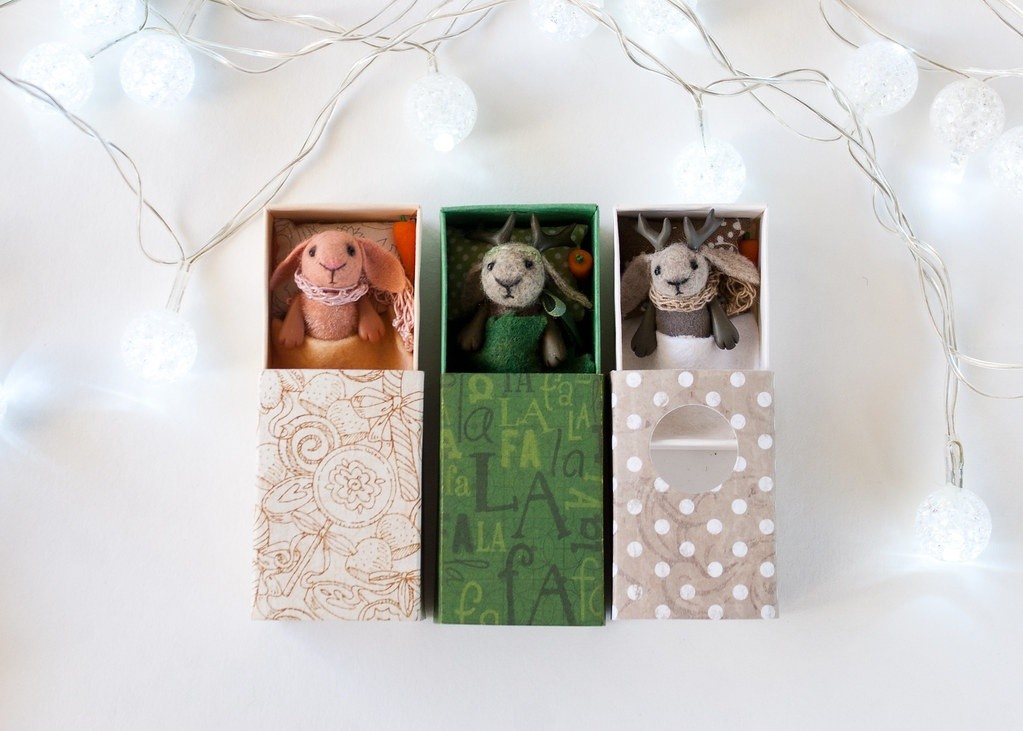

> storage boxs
[254,206,425,622]
[440,204,607,626]
[612,204,781,623]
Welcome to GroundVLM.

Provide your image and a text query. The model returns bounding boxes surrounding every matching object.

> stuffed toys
[458,211,593,376]
[270,231,409,350]
[618,208,762,359]
